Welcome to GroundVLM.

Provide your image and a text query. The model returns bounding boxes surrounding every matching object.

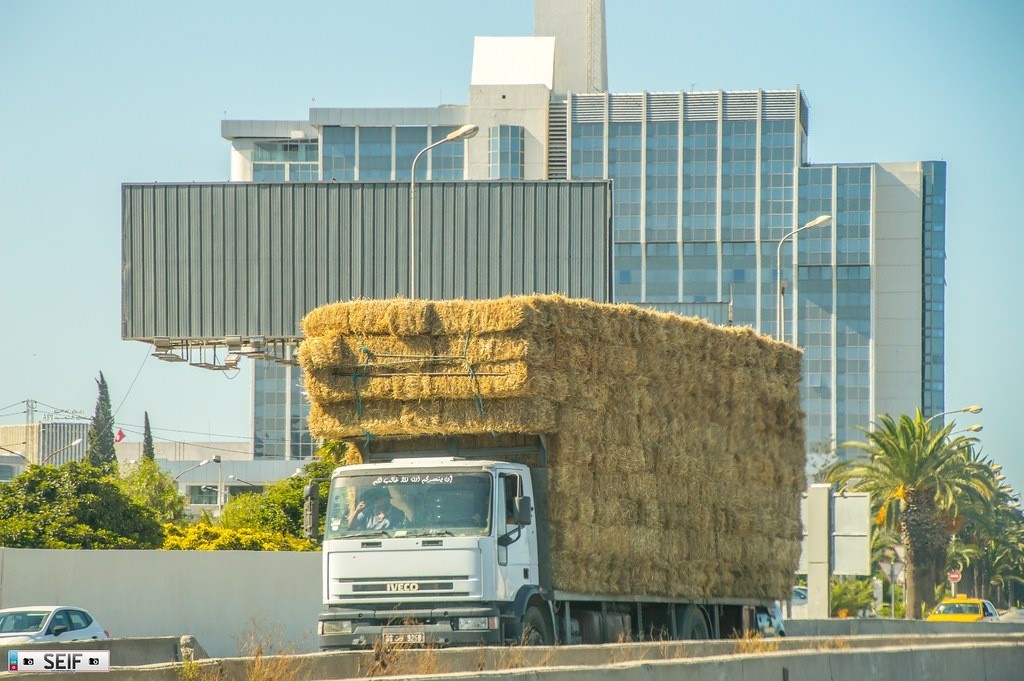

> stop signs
[947,568,962,584]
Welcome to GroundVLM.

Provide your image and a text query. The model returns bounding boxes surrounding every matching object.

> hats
[371,484,392,497]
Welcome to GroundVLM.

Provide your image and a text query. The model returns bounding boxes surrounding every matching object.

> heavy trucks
[317,429,778,651]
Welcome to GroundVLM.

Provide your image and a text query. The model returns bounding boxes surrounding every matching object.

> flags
[115,429,126,442]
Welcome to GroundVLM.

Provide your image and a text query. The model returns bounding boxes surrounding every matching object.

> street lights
[411,124,480,299]
[775,215,833,342]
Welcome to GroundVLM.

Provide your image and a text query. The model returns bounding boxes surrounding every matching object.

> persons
[347,486,410,531]
[467,489,514,526]
[859,603,875,618]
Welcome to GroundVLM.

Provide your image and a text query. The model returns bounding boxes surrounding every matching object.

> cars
[783,584,807,605]
[925,593,1001,624]
[756,600,786,637]
[0,606,111,644]
